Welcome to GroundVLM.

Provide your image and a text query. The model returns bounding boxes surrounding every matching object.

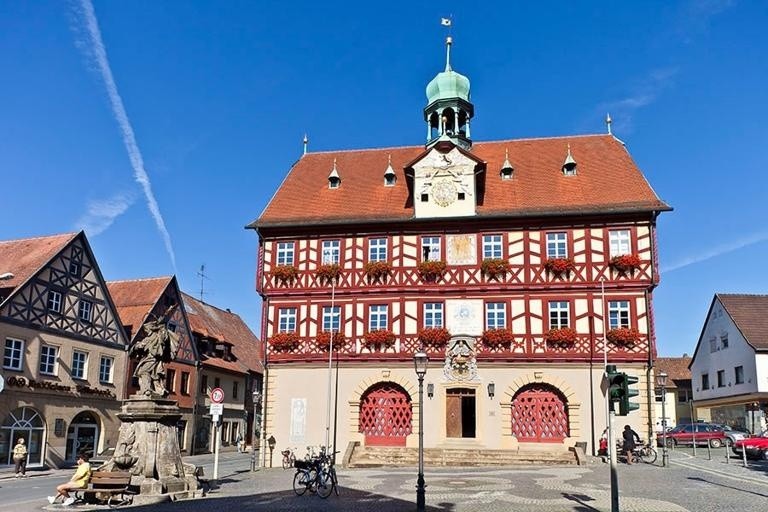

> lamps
[487,383,495,399]
[427,383,434,399]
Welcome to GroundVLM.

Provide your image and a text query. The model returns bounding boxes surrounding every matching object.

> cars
[89,447,116,471]
[657,422,768,461]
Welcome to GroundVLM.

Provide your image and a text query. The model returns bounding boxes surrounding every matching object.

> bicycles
[282,446,340,499]
[616,440,657,464]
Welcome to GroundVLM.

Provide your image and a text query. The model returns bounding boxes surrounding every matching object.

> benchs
[67,471,136,509]
[196,465,211,490]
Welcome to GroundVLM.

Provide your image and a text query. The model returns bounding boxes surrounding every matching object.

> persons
[127,321,183,396]
[622,424,642,464]
[235,432,243,454]
[48,454,92,505]
[13,437,28,477]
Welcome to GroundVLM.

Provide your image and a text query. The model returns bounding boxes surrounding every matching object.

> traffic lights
[609,372,639,416]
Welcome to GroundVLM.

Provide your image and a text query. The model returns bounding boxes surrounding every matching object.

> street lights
[413,349,430,512]
[752,400,760,434]
[252,389,260,452]
[656,369,668,466]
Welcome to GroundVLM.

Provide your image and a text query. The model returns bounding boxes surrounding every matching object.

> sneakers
[14,471,28,478]
[60,496,76,507]
[46,493,56,505]
